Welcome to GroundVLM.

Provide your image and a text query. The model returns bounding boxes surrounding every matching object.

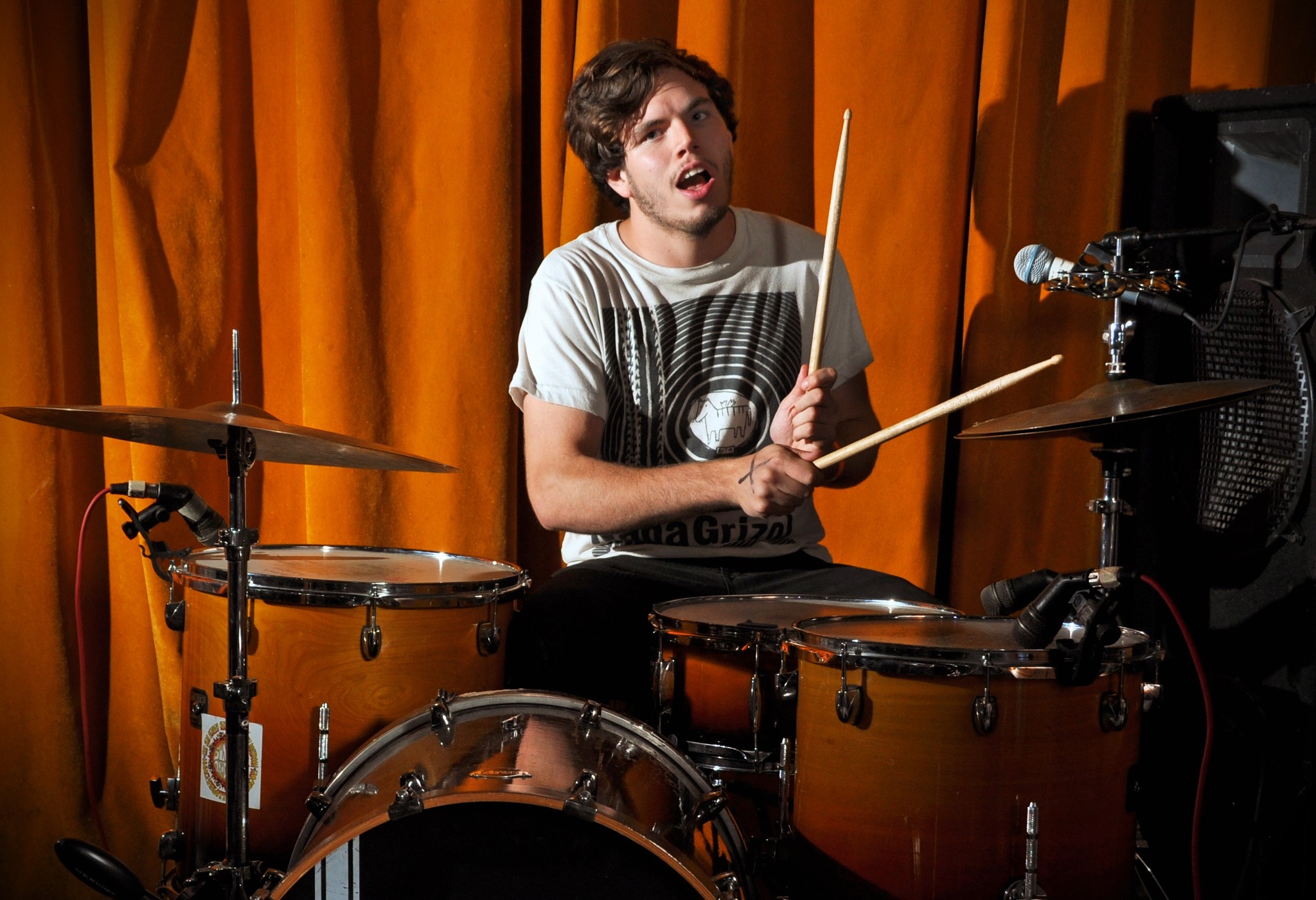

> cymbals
[2,403,457,474]
[953,377,1276,441]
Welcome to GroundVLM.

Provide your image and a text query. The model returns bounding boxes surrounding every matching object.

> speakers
[1113,85,1316,900]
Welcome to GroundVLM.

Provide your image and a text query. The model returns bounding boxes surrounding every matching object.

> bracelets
[823,442,845,480]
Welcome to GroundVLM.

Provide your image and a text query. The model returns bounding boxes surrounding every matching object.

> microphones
[980,566,1060,647]
[1013,242,1191,321]
[1011,566,1140,652]
[109,480,229,549]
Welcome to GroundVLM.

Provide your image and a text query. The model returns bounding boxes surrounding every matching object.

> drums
[650,593,956,754]
[160,546,527,887]
[786,613,1159,897]
[262,687,749,900]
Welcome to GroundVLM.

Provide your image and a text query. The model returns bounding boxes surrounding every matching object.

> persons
[508,38,923,831]
